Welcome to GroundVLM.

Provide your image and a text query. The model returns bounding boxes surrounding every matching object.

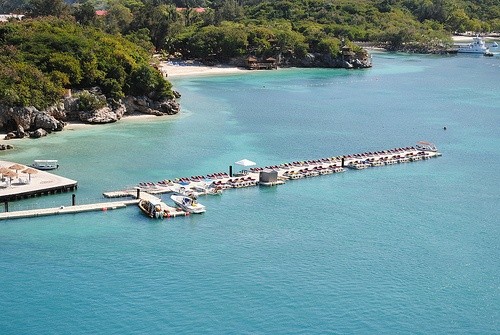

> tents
[234,158,256,175]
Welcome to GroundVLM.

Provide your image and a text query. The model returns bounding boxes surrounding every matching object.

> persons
[140,196,195,216]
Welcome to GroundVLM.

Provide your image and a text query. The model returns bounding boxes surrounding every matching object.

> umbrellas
[0,164,39,185]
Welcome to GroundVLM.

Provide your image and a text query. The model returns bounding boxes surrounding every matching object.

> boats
[459,40,500,57]
[170,195,206,214]
[30,160,58,170]
[139,197,188,217]
